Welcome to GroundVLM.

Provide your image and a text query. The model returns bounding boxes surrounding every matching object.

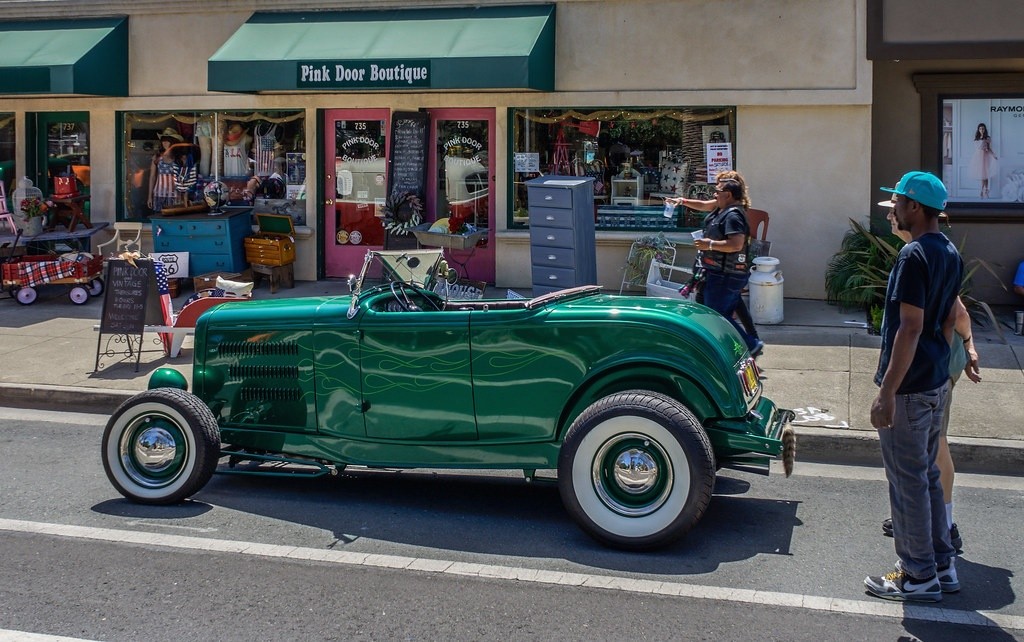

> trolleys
[0,228,105,306]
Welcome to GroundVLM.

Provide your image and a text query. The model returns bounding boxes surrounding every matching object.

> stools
[248,262,295,292]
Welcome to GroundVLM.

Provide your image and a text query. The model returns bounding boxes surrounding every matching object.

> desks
[47,195,93,233]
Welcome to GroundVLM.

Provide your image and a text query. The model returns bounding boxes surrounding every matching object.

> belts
[703,256,745,270]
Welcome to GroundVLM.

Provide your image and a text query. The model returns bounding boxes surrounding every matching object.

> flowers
[16,198,52,220]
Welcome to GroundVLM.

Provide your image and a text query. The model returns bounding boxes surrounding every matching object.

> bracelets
[709,240,714,250]
[963,336,971,343]
[683,199,687,205]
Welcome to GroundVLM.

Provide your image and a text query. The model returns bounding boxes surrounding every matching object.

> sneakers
[893,556,961,593]
[863,570,942,603]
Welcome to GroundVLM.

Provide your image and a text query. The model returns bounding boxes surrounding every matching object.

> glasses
[714,189,725,194]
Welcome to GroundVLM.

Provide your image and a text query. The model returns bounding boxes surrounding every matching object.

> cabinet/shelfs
[611,169,645,206]
[150,208,253,278]
[525,177,593,298]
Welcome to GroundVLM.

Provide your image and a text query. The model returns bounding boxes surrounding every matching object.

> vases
[21,213,41,238]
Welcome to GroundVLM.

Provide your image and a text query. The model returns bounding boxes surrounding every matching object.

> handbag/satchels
[727,239,769,276]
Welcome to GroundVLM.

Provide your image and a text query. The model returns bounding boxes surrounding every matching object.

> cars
[100,247,798,552]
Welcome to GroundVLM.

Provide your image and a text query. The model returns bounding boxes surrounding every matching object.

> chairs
[731,208,770,318]
[646,156,692,207]
[97,223,144,277]
[0,181,17,238]
[574,154,609,205]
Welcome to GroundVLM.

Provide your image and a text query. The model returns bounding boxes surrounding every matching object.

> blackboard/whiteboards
[100,259,164,333]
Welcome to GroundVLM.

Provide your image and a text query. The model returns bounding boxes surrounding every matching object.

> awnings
[0,16,129,96]
[208,3,557,95]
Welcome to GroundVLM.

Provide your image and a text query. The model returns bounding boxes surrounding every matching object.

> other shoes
[949,524,963,551]
[882,516,894,534]
[750,341,763,359]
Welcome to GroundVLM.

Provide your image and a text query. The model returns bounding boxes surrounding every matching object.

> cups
[663,202,675,218]
[690,229,704,241]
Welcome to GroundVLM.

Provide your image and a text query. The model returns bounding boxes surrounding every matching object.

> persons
[864,171,982,602]
[1013,261,1024,295]
[147,127,184,212]
[969,123,998,199]
[663,171,764,358]
[224,124,252,176]
[196,116,223,177]
[254,121,284,171]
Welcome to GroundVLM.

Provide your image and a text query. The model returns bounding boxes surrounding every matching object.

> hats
[878,182,947,218]
[157,127,183,143]
[880,171,949,210]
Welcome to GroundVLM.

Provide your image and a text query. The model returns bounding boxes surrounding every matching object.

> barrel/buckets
[1014,310,1024,335]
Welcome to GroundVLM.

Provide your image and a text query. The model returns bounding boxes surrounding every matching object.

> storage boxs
[193,271,243,294]
[243,215,298,267]
